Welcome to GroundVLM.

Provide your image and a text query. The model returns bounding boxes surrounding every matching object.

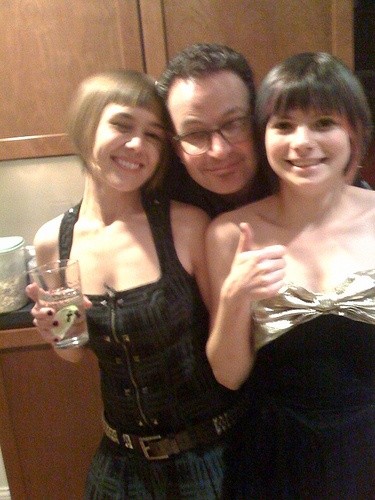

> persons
[154,41,375,219]
[204,51,375,500]
[25,66,231,500]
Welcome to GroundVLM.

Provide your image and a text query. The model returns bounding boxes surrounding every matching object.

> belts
[101,401,239,459]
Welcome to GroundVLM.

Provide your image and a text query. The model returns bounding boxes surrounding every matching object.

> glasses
[171,114,253,156]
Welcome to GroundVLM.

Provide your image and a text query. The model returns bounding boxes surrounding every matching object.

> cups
[28,259,90,349]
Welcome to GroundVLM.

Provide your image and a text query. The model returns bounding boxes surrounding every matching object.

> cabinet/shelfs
[0,0,356,161]
[1,327,107,500]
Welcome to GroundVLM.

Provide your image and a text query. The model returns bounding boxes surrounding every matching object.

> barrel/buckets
[0,236,29,314]
